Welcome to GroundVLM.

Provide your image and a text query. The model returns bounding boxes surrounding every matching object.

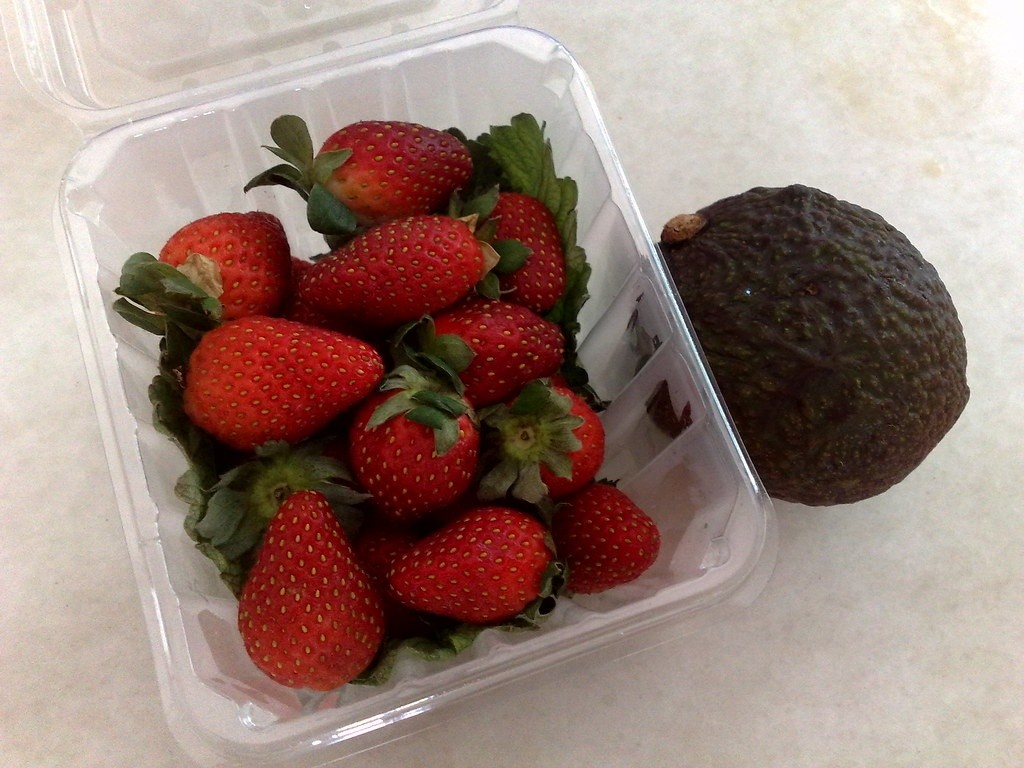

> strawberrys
[112,116,658,691]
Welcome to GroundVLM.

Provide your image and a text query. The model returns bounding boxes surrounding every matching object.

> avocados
[631,185,970,507]
